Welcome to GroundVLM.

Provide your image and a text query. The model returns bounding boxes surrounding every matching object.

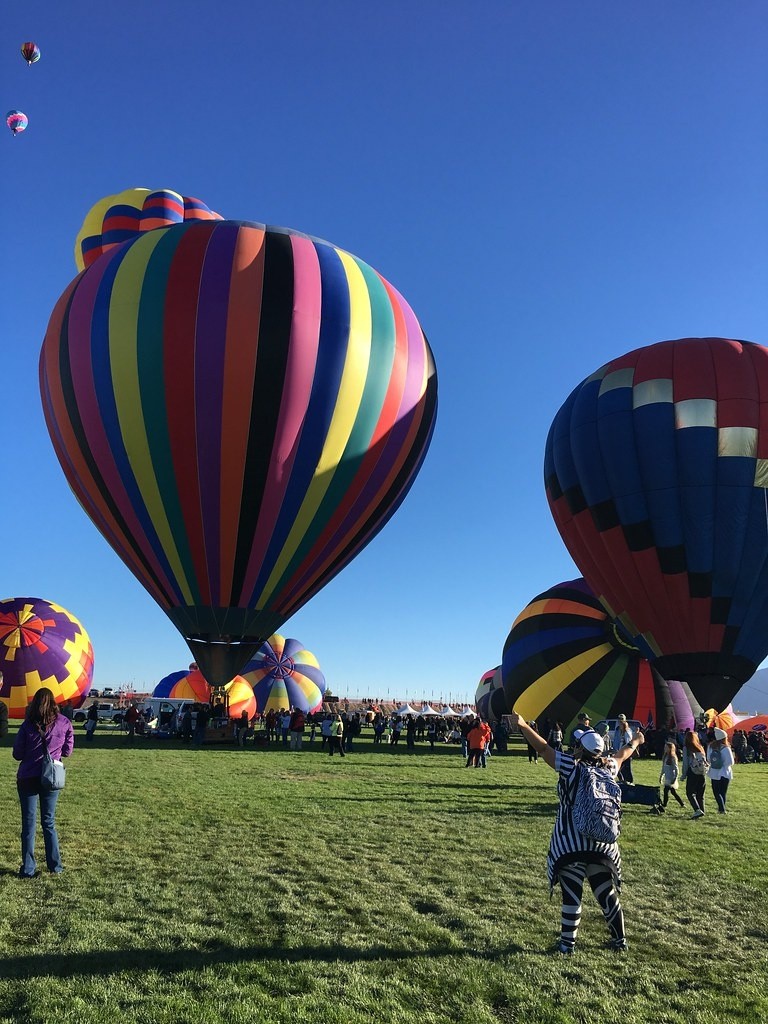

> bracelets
[627,742,636,753]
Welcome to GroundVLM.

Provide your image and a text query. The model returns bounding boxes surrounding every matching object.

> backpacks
[710,744,727,769]
[558,758,622,843]
[337,724,343,736]
[123,709,132,722]
[689,749,710,776]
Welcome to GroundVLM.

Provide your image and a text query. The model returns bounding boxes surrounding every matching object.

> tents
[395,703,478,720]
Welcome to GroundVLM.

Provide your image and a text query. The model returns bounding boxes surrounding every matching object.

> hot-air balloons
[474,665,513,723]
[700,709,768,749]
[21,41,42,68]
[500,578,695,790]
[75,188,226,280]
[242,634,327,719]
[0,598,97,718]
[541,338,768,746]
[6,109,29,137]
[151,662,257,726]
[40,217,440,746]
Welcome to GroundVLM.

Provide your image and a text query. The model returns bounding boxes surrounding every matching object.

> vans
[142,696,194,735]
[176,701,211,740]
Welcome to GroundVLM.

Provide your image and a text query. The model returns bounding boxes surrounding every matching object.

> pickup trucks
[72,703,127,724]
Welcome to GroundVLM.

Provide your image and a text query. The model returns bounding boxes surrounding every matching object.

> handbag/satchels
[41,758,66,790]
[377,724,385,733]
[85,719,96,734]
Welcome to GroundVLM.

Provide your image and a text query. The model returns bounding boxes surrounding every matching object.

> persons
[117,703,155,743]
[259,707,361,757]
[681,732,708,820]
[708,728,735,815]
[0,670,9,742]
[182,697,256,747]
[12,688,74,879]
[569,714,634,788]
[523,717,563,765]
[362,698,473,710]
[83,700,98,745]
[667,725,768,764]
[510,708,645,956]
[365,712,509,768]
[62,699,73,724]
[658,742,687,808]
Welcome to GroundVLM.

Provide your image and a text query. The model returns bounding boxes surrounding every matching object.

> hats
[574,729,605,757]
[714,727,728,740]
[93,700,100,704]
[578,713,593,721]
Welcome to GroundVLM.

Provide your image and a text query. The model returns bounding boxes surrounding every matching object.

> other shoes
[691,809,705,819]
[717,810,726,814]
